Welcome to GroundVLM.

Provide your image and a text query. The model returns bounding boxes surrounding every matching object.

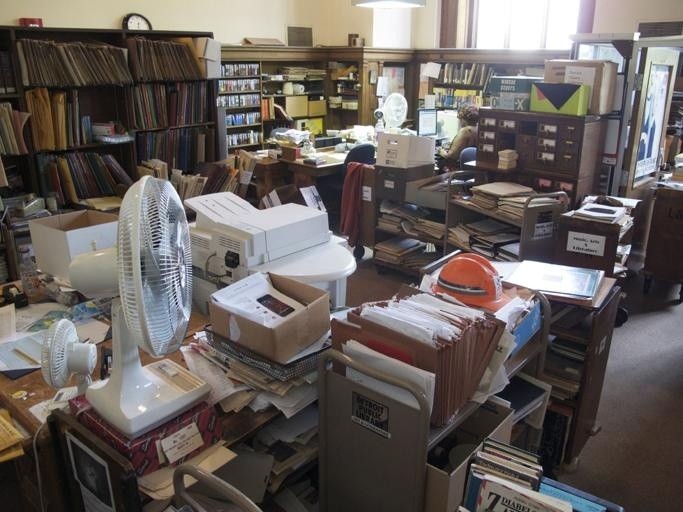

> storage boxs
[26,211,120,283]
[204,270,333,362]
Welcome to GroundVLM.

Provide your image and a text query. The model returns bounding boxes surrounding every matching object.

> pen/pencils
[14,347,41,365]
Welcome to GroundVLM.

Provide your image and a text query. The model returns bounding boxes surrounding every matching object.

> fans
[83,175,214,440]
[38,317,99,409]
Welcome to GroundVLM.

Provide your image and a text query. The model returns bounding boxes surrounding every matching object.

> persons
[438,106,478,161]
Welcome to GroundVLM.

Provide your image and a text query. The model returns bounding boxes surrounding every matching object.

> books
[218,63,328,146]
[462,338,587,512]
[433,63,489,109]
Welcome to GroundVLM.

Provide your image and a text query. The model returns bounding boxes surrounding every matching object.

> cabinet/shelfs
[416,250,621,476]
[53,366,324,512]
[317,281,551,506]
[212,39,637,208]
[361,168,641,328]
[0,22,237,281]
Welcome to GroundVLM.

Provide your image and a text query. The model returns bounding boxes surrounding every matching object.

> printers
[183,191,357,320]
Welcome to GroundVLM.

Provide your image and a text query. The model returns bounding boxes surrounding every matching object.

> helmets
[432,253,512,312]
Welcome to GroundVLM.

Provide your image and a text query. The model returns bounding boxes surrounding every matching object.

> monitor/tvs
[415,108,438,137]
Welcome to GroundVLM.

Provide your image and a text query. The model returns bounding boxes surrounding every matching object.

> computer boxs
[436,109,465,142]
